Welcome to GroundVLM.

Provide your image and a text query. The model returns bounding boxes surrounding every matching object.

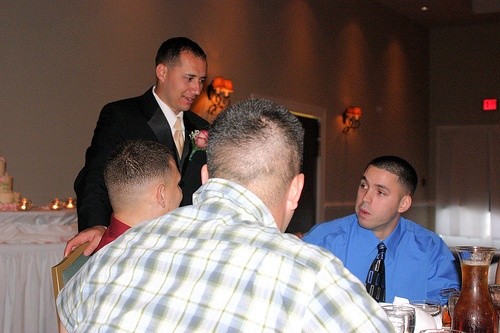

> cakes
[0,157,21,205]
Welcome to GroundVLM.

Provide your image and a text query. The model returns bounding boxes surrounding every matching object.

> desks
[0,208,79,333]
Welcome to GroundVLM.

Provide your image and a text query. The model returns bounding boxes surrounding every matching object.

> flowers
[187,129,210,161]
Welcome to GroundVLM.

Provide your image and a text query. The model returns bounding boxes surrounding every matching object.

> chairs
[51,241,93,333]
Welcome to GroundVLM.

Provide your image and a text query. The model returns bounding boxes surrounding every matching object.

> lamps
[342,106,362,136]
[207,75,234,116]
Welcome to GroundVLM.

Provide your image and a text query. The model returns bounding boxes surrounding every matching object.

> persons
[88,137,183,257]
[56,96,397,333]
[62,36,213,257]
[300,156,461,307]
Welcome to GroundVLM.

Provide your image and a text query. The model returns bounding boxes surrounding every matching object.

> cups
[380,305,416,333]
[449,291,461,320]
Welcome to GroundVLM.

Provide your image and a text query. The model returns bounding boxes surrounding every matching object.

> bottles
[452,245,500,333]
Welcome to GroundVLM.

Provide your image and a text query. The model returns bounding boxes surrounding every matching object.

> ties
[174,117,185,160]
[365,242,387,303]
